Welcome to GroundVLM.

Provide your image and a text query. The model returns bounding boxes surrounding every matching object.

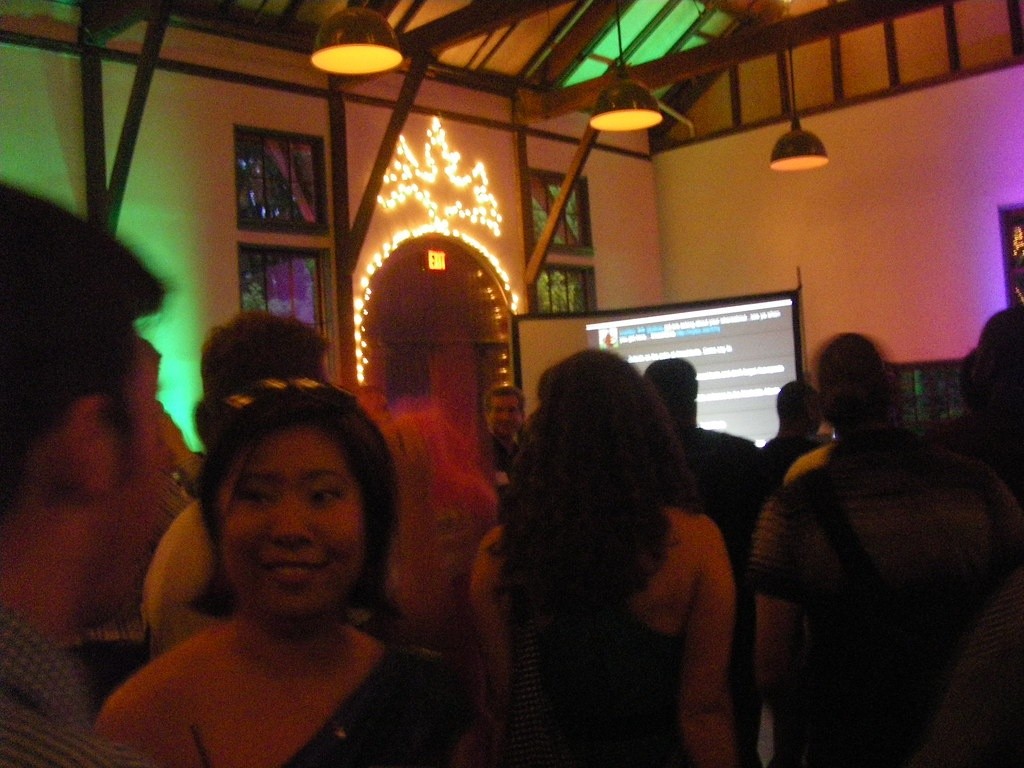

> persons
[0,183,486,768]
[644,358,776,768]
[472,350,739,768]
[487,387,527,524]
[751,305,1024,768]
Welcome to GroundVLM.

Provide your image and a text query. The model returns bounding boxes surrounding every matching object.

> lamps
[767,46,830,172]
[309,0,404,76]
[589,0,665,133]
